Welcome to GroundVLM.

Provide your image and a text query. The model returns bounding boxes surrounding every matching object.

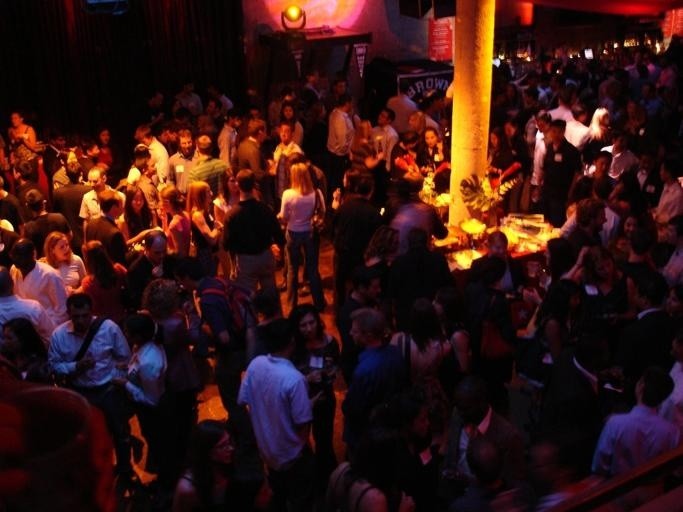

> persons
[0,31,683,512]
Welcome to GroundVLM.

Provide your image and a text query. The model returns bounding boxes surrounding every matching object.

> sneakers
[130,438,145,465]
[275,281,313,297]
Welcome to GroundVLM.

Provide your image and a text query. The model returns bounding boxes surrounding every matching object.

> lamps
[277,3,307,34]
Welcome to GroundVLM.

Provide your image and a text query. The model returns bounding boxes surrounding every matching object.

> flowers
[417,161,450,221]
[459,162,524,224]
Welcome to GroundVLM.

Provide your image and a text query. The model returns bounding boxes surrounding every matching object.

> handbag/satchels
[308,187,331,242]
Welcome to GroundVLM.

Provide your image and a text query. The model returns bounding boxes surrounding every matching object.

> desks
[431,213,563,295]
